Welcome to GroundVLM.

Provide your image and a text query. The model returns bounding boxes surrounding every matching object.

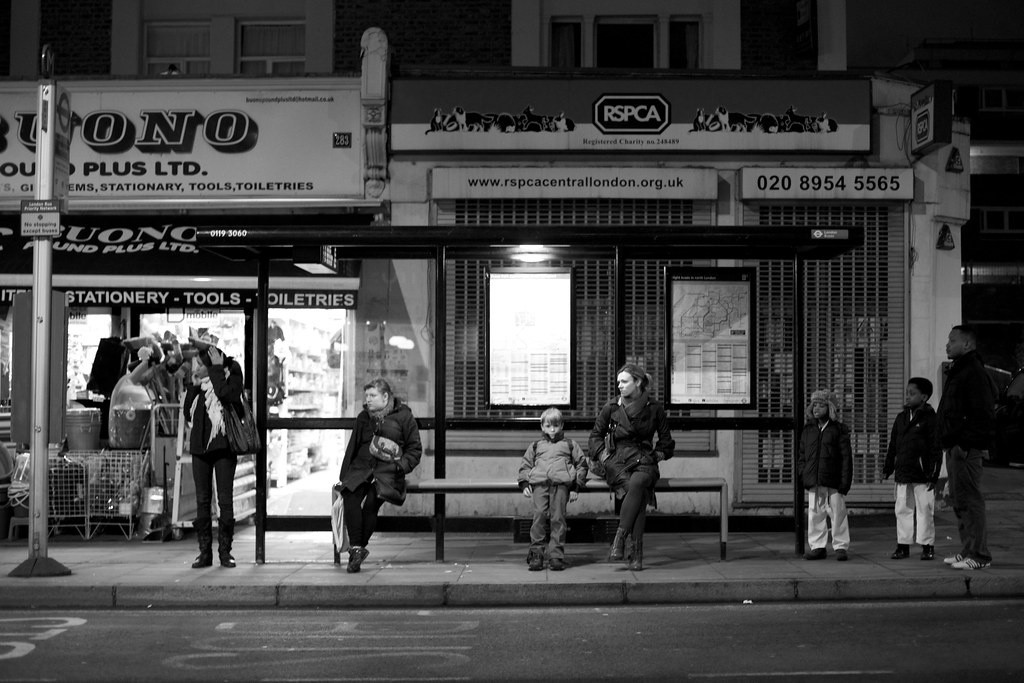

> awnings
[0,208,362,309]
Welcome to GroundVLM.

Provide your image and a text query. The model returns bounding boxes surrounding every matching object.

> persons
[937,325,994,570]
[339,379,422,572]
[518,408,589,571]
[183,345,244,566]
[883,377,942,559]
[799,389,853,560]
[587,364,675,571]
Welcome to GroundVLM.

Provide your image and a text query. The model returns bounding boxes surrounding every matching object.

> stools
[8,517,29,541]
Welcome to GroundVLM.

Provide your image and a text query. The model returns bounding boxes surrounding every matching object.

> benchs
[331,476,730,565]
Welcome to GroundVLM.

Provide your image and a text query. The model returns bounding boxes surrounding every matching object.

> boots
[217,517,236,567]
[628,540,643,571]
[191,516,213,568]
[610,527,632,560]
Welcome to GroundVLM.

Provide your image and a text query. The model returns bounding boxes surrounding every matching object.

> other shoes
[548,558,567,570]
[346,546,369,573]
[805,548,827,560]
[527,552,544,570]
[921,545,935,560]
[836,549,847,562]
[891,544,909,559]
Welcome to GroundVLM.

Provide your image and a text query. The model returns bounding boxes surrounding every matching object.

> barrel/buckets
[65,408,101,452]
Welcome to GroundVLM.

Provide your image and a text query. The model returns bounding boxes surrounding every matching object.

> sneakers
[943,554,964,564]
[951,557,991,570]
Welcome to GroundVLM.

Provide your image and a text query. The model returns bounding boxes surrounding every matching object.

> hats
[805,389,839,421]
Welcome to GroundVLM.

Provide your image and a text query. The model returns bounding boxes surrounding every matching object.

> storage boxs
[512,517,621,544]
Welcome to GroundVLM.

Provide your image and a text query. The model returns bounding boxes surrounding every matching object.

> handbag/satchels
[583,394,622,476]
[222,361,262,455]
[369,419,403,464]
[86,318,131,399]
[329,481,351,553]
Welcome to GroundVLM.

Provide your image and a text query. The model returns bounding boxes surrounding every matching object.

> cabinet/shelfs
[171,390,256,540]
[268,316,340,489]
